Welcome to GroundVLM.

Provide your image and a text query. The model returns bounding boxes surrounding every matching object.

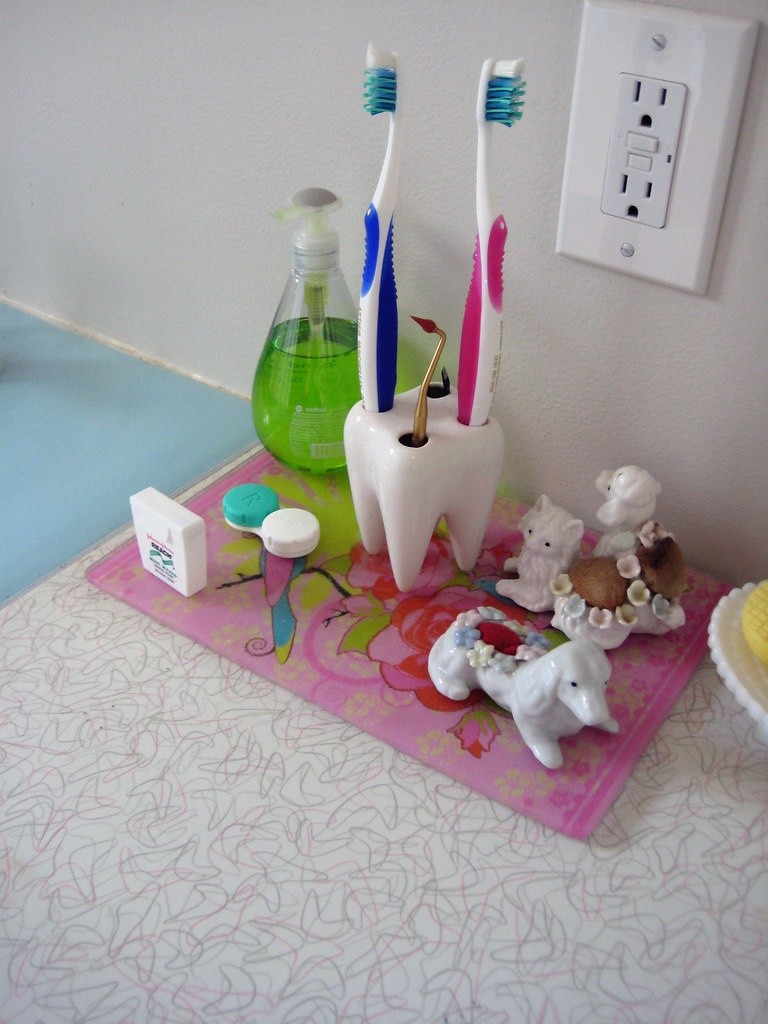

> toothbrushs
[456,56,527,426]
[356,43,408,414]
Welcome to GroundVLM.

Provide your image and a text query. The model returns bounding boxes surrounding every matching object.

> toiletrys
[246,187,376,473]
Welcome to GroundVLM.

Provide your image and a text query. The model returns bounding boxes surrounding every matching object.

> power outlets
[555,1,763,297]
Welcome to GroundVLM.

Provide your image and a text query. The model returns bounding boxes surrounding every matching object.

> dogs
[427,465,687,769]
